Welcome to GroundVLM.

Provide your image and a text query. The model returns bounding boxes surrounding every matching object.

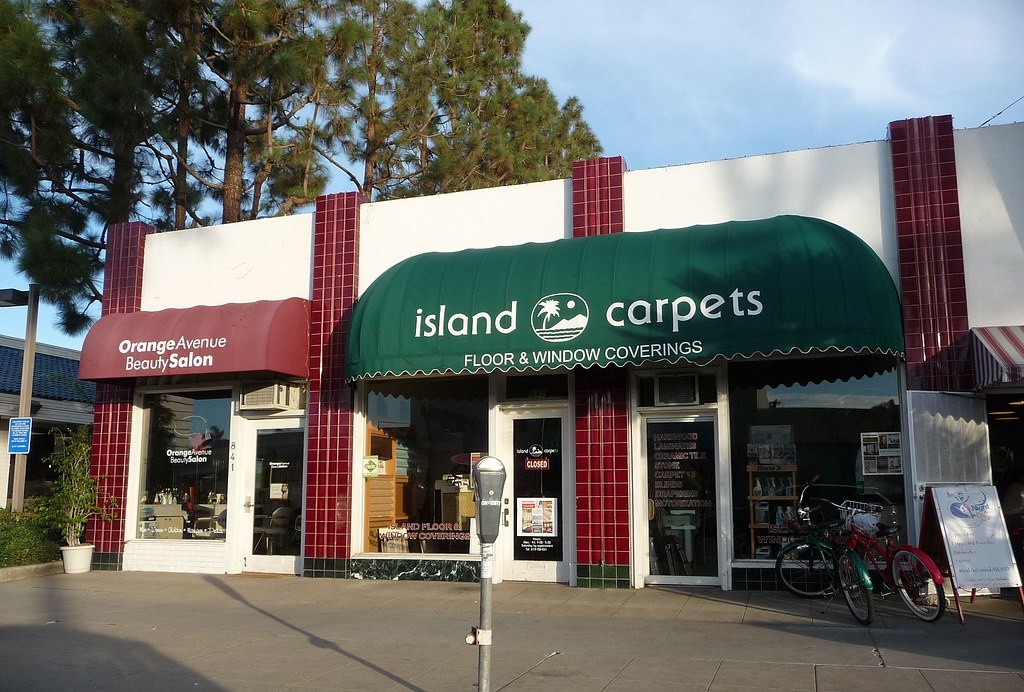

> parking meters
[472,456,508,692]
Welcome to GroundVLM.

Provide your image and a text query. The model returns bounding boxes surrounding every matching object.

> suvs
[799,478,904,536]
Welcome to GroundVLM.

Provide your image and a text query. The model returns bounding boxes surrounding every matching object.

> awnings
[78,297,310,383]
[346,219,908,387]
[971,325,1024,392]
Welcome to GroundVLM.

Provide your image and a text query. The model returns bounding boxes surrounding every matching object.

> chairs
[254,507,297,555]
[194,509,227,540]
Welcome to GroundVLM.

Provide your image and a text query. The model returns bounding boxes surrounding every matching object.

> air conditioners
[239,379,300,411]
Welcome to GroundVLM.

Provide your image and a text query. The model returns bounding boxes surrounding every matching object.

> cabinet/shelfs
[746,461,799,559]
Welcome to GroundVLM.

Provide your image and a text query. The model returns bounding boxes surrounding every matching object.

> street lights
[0,282,42,517]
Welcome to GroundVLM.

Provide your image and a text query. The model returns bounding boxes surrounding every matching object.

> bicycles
[773,476,947,624]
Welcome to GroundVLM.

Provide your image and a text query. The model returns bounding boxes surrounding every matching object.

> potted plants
[41,423,119,574]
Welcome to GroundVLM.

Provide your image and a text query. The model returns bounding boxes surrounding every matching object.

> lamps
[30,401,43,414]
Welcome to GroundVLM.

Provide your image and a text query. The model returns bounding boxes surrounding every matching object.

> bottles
[786,506,792,519]
[172,496,177,506]
[776,506,784,526]
[167,491,172,505]
[755,501,771,525]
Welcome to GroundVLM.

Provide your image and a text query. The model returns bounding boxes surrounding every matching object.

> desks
[193,504,227,540]
[140,504,184,539]
